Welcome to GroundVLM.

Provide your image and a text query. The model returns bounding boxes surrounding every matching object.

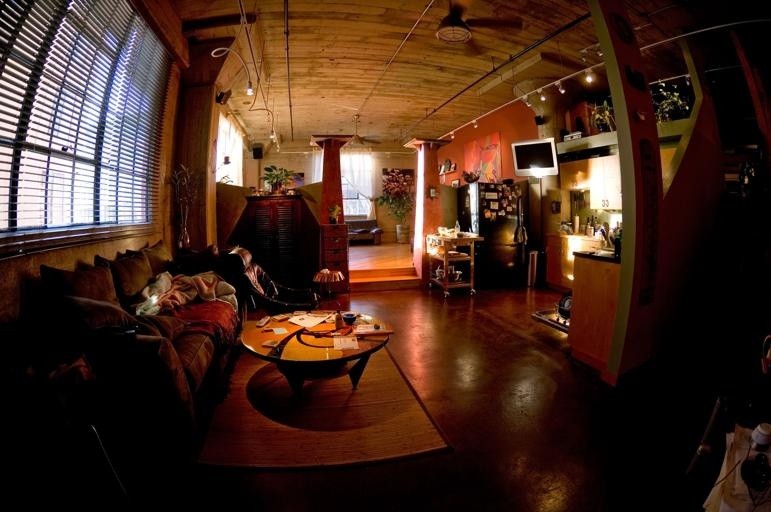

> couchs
[34,254,244,431]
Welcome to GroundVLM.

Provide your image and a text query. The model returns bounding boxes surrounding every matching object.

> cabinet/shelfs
[588,154,622,211]
[558,158,590,193]
[243,196,303,277]
[424,232,476,300]
[319,222,350,294]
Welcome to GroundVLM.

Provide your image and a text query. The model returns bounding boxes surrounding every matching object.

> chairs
[227,247,318,316]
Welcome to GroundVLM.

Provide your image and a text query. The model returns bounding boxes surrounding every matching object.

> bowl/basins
[440,272,463,281]
[462,174,481,183]
[438,226,447,236]
[343,314,356,324]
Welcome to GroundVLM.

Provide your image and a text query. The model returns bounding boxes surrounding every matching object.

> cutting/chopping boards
[447,253,468,258]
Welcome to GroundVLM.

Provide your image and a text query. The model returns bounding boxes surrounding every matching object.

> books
[354,324,396,336]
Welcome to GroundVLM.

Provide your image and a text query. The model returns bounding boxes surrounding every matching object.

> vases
[394,223,409,243]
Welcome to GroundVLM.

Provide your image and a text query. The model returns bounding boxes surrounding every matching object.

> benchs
[344,219,383,246]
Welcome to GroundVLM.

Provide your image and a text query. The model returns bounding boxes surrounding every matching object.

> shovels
[603,222,609,247]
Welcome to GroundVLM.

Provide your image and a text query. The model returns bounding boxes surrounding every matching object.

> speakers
[253,144,263,159]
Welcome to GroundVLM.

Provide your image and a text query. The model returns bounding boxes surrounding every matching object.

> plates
[443,159,451,172]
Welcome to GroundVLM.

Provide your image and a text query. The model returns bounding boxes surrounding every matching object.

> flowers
[375,168,415,224]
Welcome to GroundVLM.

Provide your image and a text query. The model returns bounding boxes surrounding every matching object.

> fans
[399,0,523,58]
[347,115,383,146]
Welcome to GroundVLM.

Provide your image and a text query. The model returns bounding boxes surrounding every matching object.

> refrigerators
[456,182,529,289]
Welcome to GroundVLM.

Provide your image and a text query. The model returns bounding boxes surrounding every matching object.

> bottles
[336,310,343,329]
[566,215,599,235]
[454,219,460,234]
[614,234,622,256]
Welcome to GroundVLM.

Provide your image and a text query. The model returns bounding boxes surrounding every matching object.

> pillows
[94,248,152,300]
[143,239,174,273]
[62,294,191,345]
[40,258,118,305]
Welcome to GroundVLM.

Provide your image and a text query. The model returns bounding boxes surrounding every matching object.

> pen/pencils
[314,315,327,317]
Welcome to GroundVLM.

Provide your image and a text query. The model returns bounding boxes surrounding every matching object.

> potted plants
[163,163,202,248]
[259,161,297,195]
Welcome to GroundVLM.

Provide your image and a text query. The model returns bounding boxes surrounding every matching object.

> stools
[312,269,344,311]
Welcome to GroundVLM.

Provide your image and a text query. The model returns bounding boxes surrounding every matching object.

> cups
[247,186,268,196]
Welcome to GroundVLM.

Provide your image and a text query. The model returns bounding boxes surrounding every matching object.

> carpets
[184,343,453,469]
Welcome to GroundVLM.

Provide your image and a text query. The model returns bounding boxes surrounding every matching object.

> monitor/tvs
[511,137,559,176]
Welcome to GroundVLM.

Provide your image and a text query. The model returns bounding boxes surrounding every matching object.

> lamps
[543,235,603,294]
[211,13,281,154]
[450,68,597,140]
[578,33,603,64]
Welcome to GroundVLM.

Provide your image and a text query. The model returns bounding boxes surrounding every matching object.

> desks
[239,307,390,404]
